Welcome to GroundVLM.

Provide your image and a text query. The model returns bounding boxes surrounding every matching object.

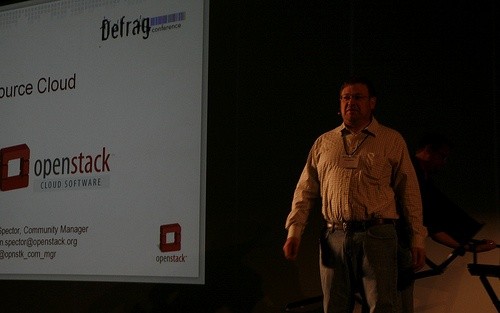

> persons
[282,78,428,313]
[362,136,496,313]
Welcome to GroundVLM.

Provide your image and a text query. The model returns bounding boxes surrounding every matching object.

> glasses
[338,95,369,101]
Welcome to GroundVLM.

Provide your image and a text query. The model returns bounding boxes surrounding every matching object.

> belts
[325,218,394,232]
